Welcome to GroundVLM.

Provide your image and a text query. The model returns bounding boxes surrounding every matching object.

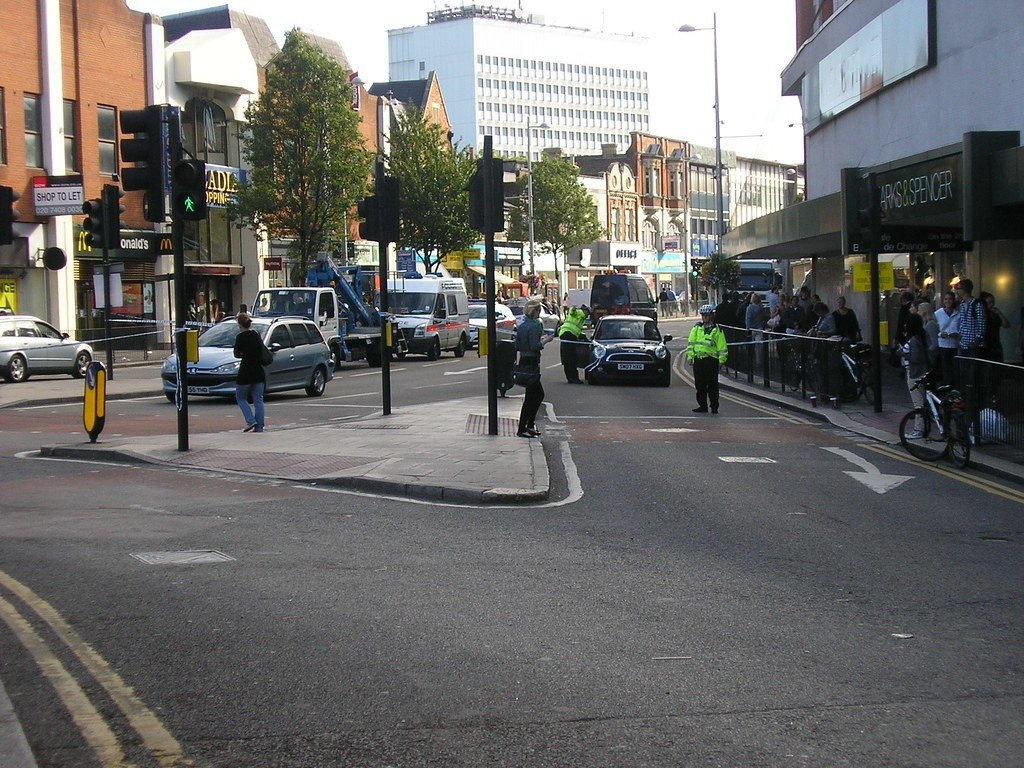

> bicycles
[784,325,875,403]
[898,368,971,469]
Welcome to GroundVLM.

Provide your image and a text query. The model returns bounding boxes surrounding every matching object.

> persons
[832,296,862,345]
[678,289,692,314]
[515,300,554,438]
[950,262,965,290]
[716,292,741,363]
[734,292,764,367]
[894,279,1010,440]
[551,299,559,314]
[495,290,504,301]
[769,285,779,311]
[542,297,553,311]
[497,297,502,303]
[234,312,266,433]
[667,288,677,316]
[236,304,251,315]
[770,286,836,356]
[687,305,728,414]
[559,303,593,383]
[923,265,936,295]
[259,298,268,312]
[659,288,669,316]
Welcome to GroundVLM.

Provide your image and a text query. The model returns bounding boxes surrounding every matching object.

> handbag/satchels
[260,346,273,366]
[767,305,781,326]
[511,365,541,387]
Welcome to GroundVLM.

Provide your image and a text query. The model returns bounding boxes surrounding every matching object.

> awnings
[467,267,528,288]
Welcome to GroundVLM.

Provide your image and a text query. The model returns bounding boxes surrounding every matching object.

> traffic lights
[171,158,206,222]
[462,174,482,228]
[81,199,105,248]
[0,184,21,246]
[358,197,380,241]
[101,184,127,249]
[119,104,164,223]
[692,270,698,277]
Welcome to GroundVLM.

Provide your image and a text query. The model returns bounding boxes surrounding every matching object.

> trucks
[374,277,471,361]
[728,259,775,309]
[250,258,409,367]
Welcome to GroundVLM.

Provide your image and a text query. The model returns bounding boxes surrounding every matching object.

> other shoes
[244,421,257,432]
[252,427,263,432]
[692,406,708,412]
[527,428,541,435]
[904,431,923,439]
[518,432,534,438]
[711,409,718,413]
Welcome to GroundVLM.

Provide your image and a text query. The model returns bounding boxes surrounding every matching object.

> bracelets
[996,312,1001,315]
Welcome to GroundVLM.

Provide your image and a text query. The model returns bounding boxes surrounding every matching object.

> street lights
[518,117,550,293]
[677,11,722,269]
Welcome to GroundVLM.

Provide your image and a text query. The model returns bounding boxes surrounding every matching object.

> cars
[1,314,93,383]
[469,305,518,349]
[585,315,673,387]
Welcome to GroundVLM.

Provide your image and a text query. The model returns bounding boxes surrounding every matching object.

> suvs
[161,315,335,404]
[504,301,561,338]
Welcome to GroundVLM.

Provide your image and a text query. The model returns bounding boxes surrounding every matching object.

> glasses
[957,287,962,289]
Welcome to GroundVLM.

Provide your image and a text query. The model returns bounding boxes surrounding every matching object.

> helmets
[699,305,712,315]
[583,303,592,314]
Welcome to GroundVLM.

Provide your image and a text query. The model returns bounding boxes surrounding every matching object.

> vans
[589,273,660,328]
